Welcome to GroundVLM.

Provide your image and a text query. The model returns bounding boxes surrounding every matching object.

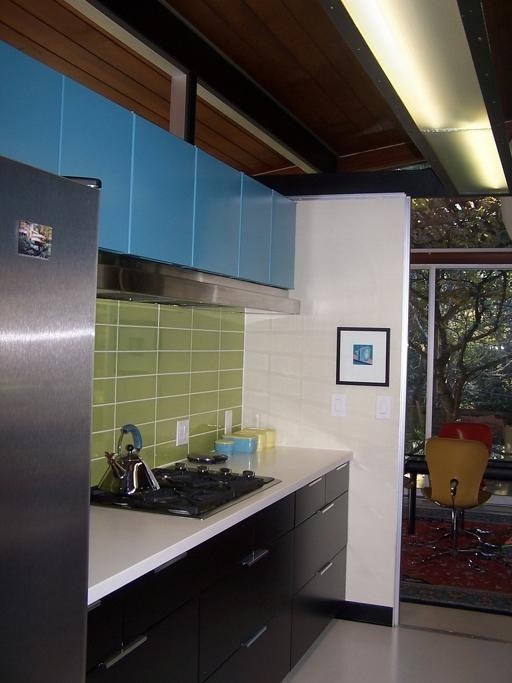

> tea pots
[99,425,160,494]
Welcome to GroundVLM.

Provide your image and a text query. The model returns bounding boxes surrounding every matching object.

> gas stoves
[89,462,282,521]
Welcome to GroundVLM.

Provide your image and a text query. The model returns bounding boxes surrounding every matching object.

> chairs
[423,423,500,549]
[409,437,497,575]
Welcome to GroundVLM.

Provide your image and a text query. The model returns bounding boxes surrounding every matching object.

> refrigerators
[0,157,102,683]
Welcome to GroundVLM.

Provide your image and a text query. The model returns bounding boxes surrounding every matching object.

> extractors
[96,249,301,318]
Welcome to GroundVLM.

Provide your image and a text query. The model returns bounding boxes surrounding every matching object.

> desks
[404,438,512,535]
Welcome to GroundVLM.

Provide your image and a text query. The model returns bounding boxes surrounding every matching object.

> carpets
[399,494,512,616]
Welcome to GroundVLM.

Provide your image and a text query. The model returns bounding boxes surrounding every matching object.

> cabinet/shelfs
[1,42,132,255]
[134,112,242,280]
[242,173,296,289]
[85,460,350,683]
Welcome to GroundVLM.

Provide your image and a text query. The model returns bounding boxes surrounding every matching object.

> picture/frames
[335,326,390,387]
